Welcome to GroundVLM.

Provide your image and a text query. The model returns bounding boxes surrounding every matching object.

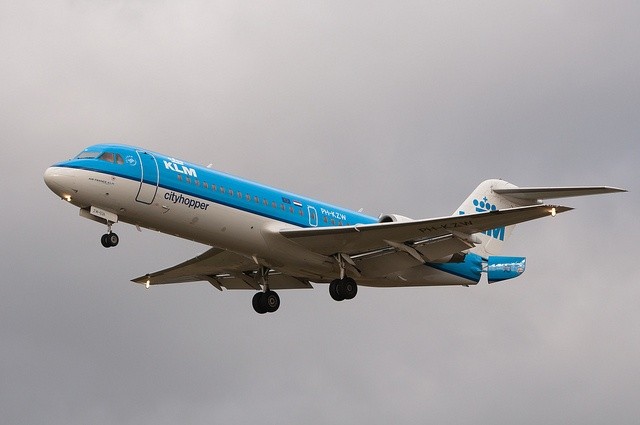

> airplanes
[44,144,630,314]
